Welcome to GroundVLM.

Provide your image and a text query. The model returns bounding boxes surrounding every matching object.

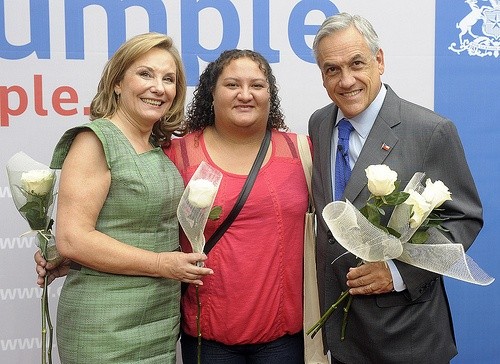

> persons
[32,46,320,363]
[49,31,216,362]
[307,11,485,363]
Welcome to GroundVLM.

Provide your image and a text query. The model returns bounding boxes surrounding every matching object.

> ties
[335,120,354,201]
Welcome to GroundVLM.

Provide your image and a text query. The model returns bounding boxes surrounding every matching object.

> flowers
[306,164,496,341]
[4,150,60,364]
[177,160,223,364]
